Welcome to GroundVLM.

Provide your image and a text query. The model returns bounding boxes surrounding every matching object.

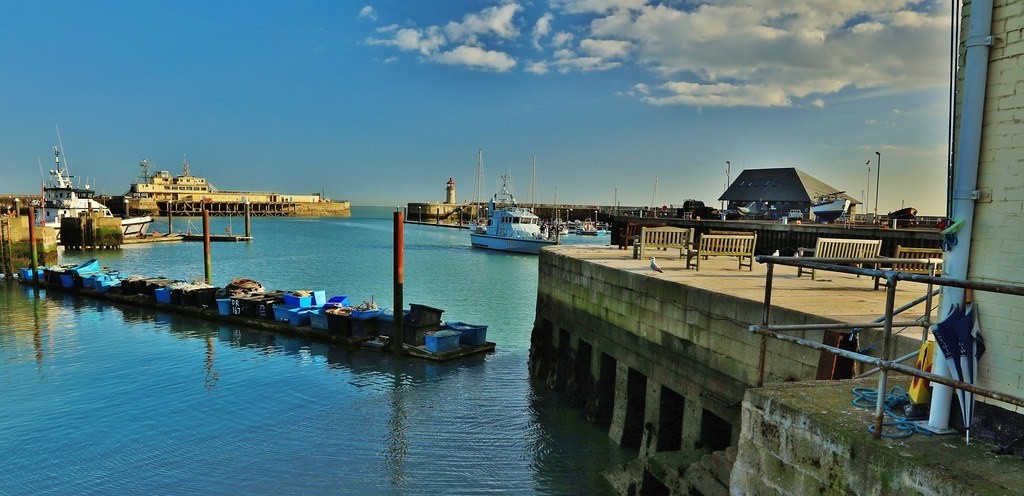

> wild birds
[793,248,804,257]
[772,250,779,257]
[650,256,664,274]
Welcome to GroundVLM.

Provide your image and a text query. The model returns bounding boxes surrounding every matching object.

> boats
[544,219,612,236]
[812,199,851,223]
[470,172,556,255]
[888,208,918,227]
[32,121,154,243]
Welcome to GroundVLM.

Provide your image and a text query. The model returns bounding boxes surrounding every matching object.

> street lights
[866,160,870,213]
[875,152,880,224]
[726,161,730,208]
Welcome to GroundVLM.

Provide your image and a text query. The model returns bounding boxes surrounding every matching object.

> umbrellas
[931,287,985,445]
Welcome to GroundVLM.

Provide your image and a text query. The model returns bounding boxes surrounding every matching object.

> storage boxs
[376,308,409,337]
[326,308,387,339]
[231,288,351,329]
[424,329,461,354]
[446,321,489,346]
[401,303,447,346]
[19,258,220,310]
[215,298,232,316]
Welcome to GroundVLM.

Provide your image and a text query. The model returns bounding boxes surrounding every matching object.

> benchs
[797,237,883,280]
[619,222,669,251]
[685,233,758,271]
[696,229,758,260]
[879,244,943,291]
[633,226,695,260]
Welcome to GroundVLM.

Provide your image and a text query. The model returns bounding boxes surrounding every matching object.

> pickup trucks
[788,210,803,222]
